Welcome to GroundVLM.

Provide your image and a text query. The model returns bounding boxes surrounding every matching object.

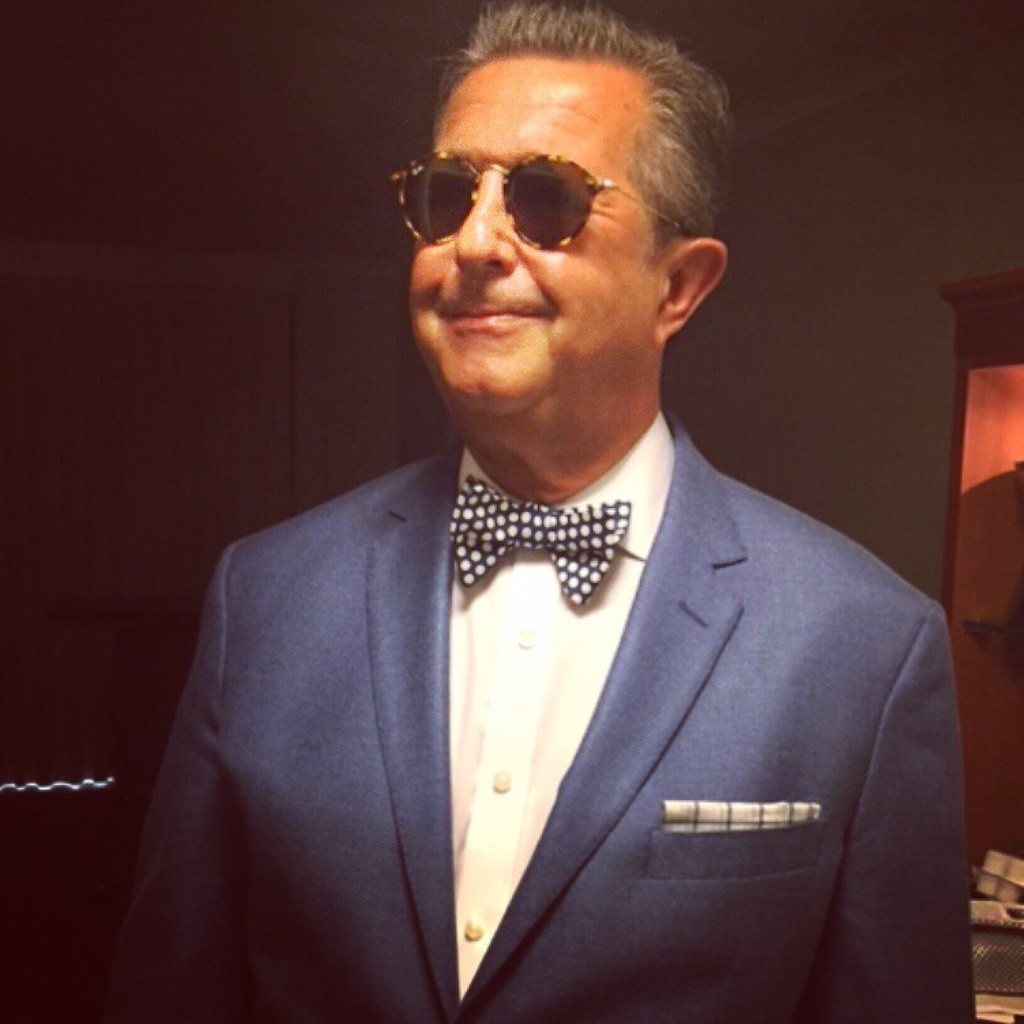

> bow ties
[450,473,631,606]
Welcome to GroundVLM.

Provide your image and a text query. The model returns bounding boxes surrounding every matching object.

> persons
[102,1,976,1024]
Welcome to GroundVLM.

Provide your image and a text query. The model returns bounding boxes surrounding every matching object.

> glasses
[392,150,680,252]
